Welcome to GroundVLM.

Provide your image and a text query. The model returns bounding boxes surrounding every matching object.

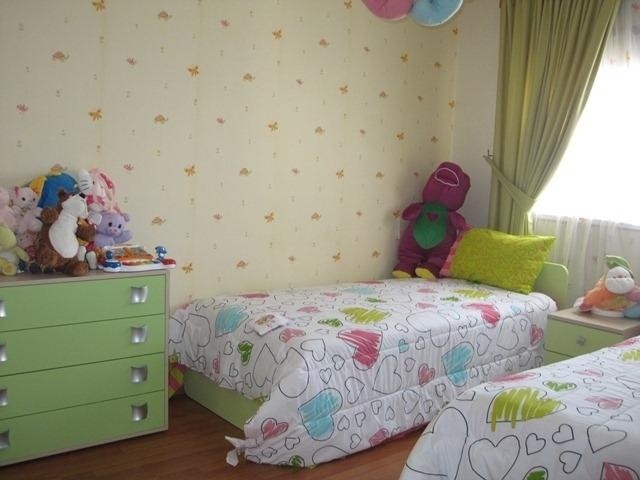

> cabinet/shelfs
[0,268,169,468]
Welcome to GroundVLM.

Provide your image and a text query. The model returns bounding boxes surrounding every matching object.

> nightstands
[543,306,640,365]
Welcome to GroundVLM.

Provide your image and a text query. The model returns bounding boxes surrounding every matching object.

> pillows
[450,228,556,295]
[440,225,472,278]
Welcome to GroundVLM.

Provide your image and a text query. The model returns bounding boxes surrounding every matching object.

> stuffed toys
[391,160,470,285]
[0,164,135,272]
[571,252,640,323]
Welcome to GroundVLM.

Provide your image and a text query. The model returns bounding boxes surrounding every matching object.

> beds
[398,333,640,480]
[169,261,569,470]
